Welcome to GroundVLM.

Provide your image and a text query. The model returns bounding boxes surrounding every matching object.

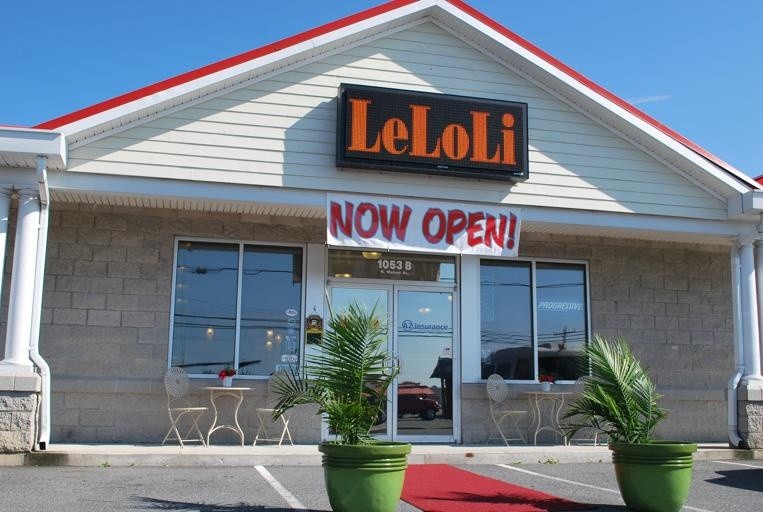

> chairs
[157,366,294,451]
[483,371,608,448]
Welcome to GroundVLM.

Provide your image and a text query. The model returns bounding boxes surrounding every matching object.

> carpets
[405,463,579,512]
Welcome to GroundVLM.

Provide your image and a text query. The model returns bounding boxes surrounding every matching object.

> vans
[490,342,586,380]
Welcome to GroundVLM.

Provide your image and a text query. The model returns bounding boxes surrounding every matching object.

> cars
[398,393,441,421]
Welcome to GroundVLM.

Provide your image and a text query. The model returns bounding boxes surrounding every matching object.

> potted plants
[269,295,416,510]
[560,328,695,510]
